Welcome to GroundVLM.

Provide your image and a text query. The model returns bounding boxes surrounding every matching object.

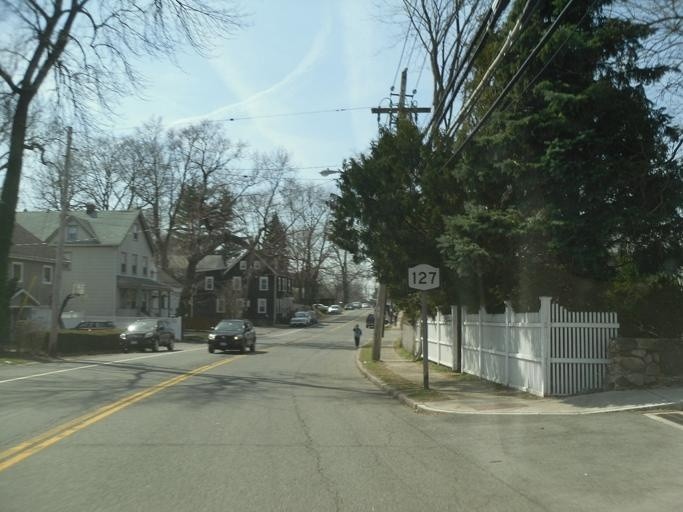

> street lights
[318,167,380,194]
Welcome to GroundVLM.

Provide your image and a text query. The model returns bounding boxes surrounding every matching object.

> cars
[207,318,256,353]
[362,303,368,308]
[365,314,374,328]
[290,312,311,327]
[328,305,340,314]
[311,304,327,311]
[119,319,175,353]
[344,303,353,310]
[352,302,360,309]
[69,320,114,331]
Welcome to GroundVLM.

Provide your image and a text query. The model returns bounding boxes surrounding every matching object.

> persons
[353,324,362,346]
